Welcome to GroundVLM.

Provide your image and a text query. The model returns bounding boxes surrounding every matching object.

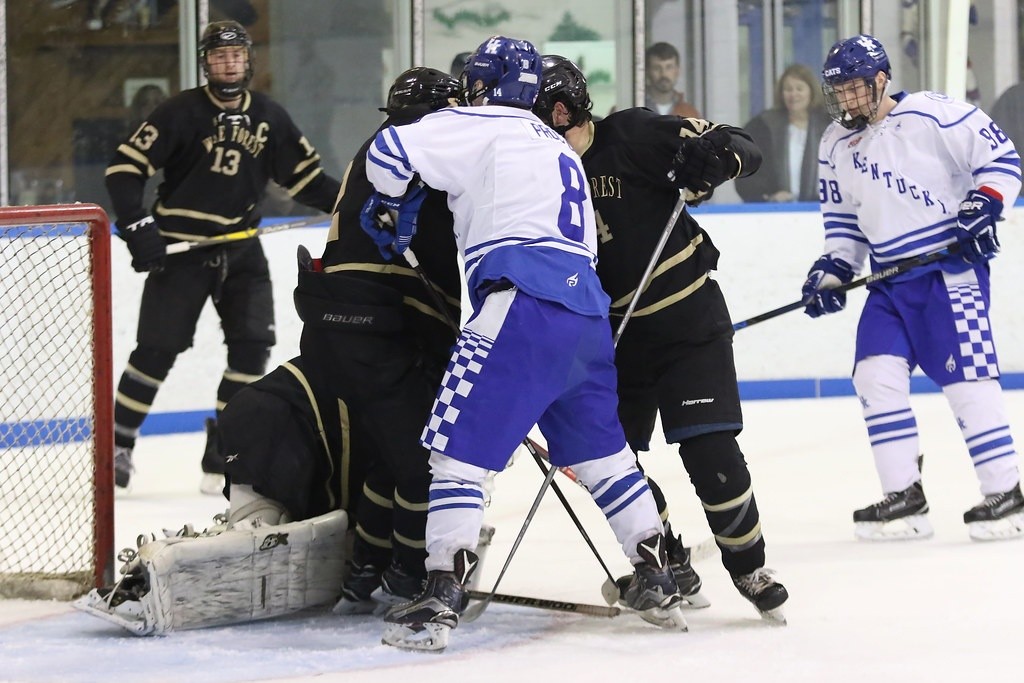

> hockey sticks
[163,209,336,256]
[468,588,620,617]
[372,203,620,606]
[457,189,691,624]
[522,435,580,482]
[734,239,967,329]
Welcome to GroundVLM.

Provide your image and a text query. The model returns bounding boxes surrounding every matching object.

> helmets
[198,20,257,98]
[819,34,891,131]
[386,65,462,119]
[536,52,587,115]
[458,34,544,112]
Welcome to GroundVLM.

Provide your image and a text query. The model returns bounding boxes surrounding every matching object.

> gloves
[670,139,721,193]
[116,212,166,272]
[358,180,426,263]
[802,253,855,317]
[957,191,1001,267]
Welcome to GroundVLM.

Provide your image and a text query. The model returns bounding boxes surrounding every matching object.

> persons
[611,43,700,123]
[104,16,343,488]
[733,61,835,204]
[218,354,350,523]
[366,35,683,630]
[78,84,169,219]
[8,43,75,207]
[529,55,789,612]
[803,35,1024,522]
[322,64,428,608]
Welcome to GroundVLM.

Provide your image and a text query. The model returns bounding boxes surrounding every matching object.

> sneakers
[853,453,934,541]
[964,481,1024,542]
[729,565,789,626]
[615,532,690,633]
[666,534,710,608]
[114,442,133,490]
[369,547,427,616]
[380,545,477,655]
[329,546,387,618]
